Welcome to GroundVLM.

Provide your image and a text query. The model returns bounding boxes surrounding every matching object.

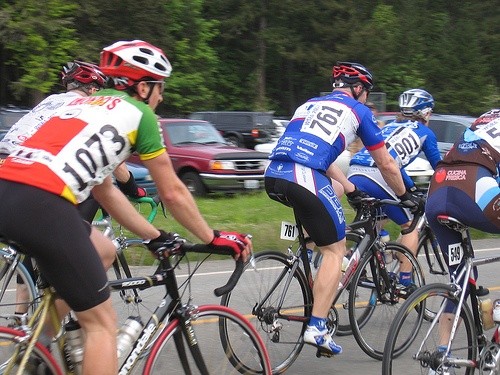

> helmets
[100,39,172,90]
[61,60,108,89]
[332,60,373,92]
[398,89,435,116]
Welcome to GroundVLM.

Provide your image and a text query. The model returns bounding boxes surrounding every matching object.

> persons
[264,62,427,354]
[0,40,252,375]
[428,109,500,375]
[347,89,443,288]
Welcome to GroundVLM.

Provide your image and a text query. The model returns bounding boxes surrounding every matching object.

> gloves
[395,190,425,216]
[345,185,370,212]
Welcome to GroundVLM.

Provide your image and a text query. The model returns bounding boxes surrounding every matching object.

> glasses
[136,81,166,93]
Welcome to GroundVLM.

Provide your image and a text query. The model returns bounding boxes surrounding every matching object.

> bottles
[380,229,395,264]
[476,285,495,331]
[62,317,84,375]
[342,253,351,276]
[117,314,144,369]
[310,245,323,280]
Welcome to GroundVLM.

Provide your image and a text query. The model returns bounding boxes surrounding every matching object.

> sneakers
[303,324,342,354]
[301,248,313,264]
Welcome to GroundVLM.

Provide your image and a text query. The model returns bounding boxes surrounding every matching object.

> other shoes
[7,313,27,329]
[428,366,450,375]
[15,344,53,375]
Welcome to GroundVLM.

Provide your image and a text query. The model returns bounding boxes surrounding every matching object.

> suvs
[186,111,276,147]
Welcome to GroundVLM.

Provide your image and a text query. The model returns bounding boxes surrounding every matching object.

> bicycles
[0,195,500,375]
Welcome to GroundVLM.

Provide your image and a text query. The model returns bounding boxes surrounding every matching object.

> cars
[129,116,269,196]
[0,109,157,195]
[256,119,434,192]
[373,111,480,158]
